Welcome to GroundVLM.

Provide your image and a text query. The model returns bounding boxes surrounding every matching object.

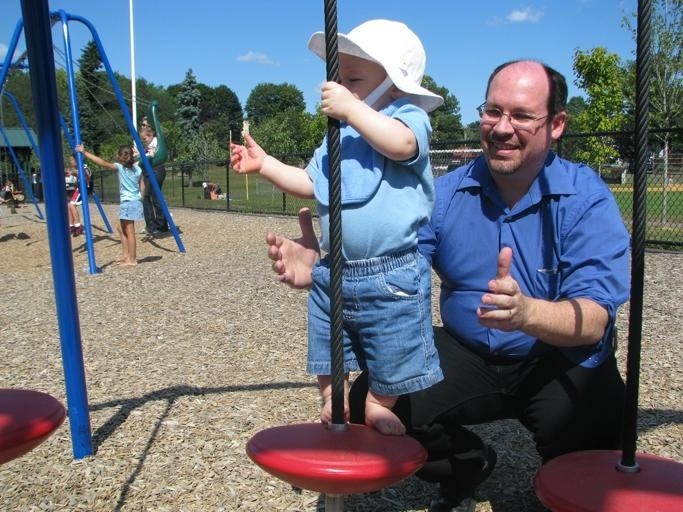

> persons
[231,14,451,436]
[2,117,167,268]
[265,56,631,511]
[203,182,221,200]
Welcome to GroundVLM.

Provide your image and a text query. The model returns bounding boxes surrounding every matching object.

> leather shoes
[431,446,497,511]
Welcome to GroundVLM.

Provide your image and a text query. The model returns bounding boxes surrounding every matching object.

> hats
[307,18,444,112]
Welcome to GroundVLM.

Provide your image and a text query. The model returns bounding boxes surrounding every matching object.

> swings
[22,42,167,199]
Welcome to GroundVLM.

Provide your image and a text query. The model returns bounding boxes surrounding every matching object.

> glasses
[476,101,549,127]
